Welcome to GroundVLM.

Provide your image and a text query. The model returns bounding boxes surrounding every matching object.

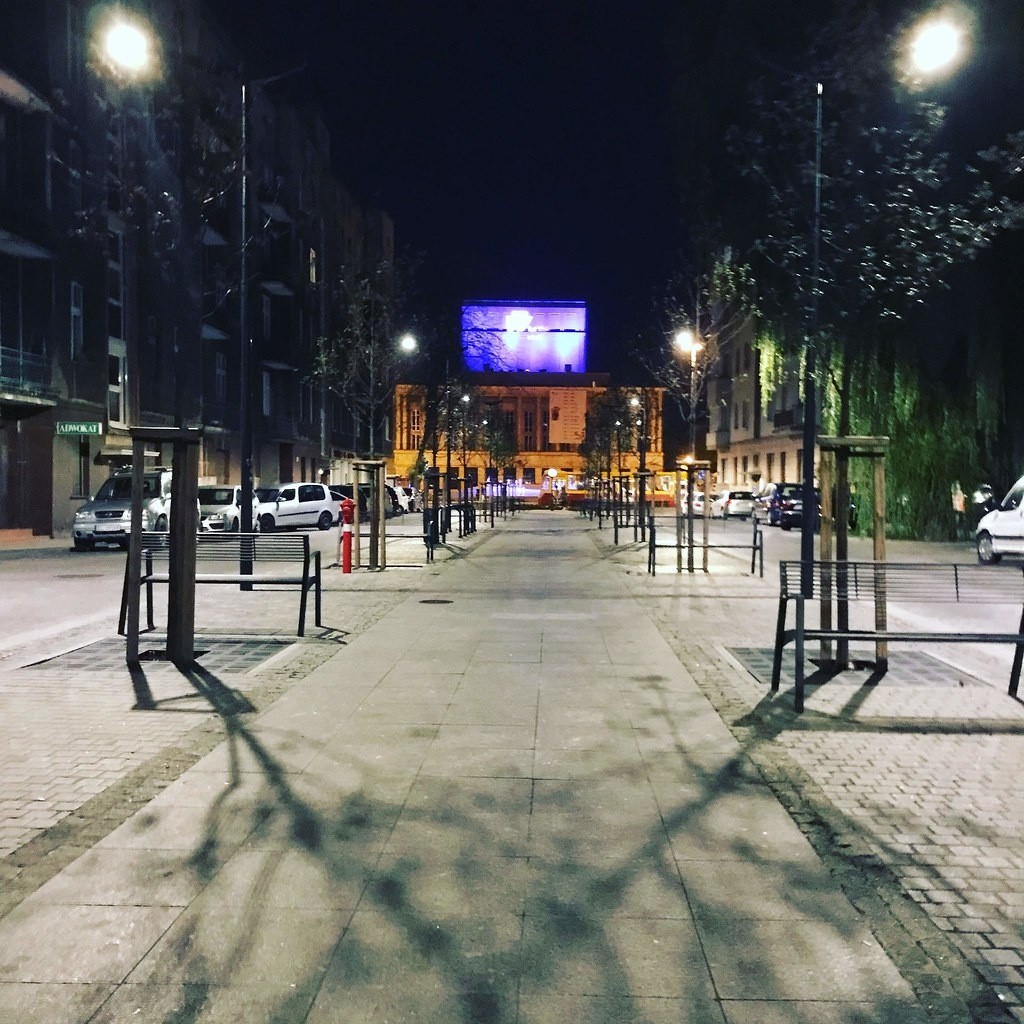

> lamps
[483,364,573,373]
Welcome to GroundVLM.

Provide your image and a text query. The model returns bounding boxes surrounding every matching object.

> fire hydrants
[339,496,355,575]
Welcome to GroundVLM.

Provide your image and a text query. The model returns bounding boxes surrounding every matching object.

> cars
[254,482,422,533]
[778,488,856,530]
[974,475,1023,566]
[680,490,756,521]
[198,485,260,536]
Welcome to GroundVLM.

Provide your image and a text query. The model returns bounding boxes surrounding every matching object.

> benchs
[116,524,322,637]
[771,560,1024,713]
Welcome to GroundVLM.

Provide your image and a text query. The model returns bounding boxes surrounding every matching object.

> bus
[538,471,697,511]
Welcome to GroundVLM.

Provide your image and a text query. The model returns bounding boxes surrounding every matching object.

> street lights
[631,388,647,541]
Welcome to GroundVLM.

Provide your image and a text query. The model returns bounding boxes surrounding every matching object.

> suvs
[748,482,803,526]
[71,465,201,553]
